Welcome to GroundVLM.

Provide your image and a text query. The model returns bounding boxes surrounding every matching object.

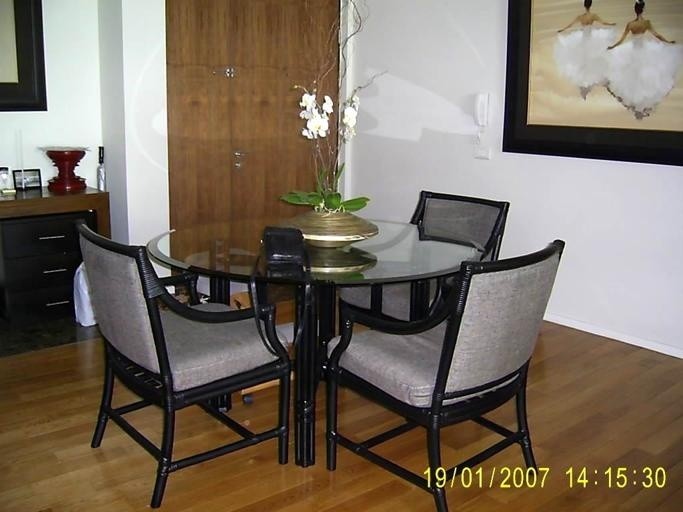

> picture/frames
[13,169,42,190]
[0,0,48,111]
[502,0,683,168]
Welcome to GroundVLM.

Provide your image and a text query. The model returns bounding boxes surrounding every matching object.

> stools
[185,250,293,405]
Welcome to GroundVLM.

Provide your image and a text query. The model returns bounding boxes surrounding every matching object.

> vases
[281,212,378,248]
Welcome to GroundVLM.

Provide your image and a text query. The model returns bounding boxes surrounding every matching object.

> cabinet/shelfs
[165,0,340,264]
[0,193,110,356]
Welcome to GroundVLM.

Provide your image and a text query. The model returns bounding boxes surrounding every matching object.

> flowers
[280,0,370,214]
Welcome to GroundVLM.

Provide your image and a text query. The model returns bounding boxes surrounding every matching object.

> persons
[603,0,680,119]
[555,0,616,100]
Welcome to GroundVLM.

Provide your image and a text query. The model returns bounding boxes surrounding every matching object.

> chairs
[327,240,567,512]
[339,190,510,335]
[79,225,292,509]
